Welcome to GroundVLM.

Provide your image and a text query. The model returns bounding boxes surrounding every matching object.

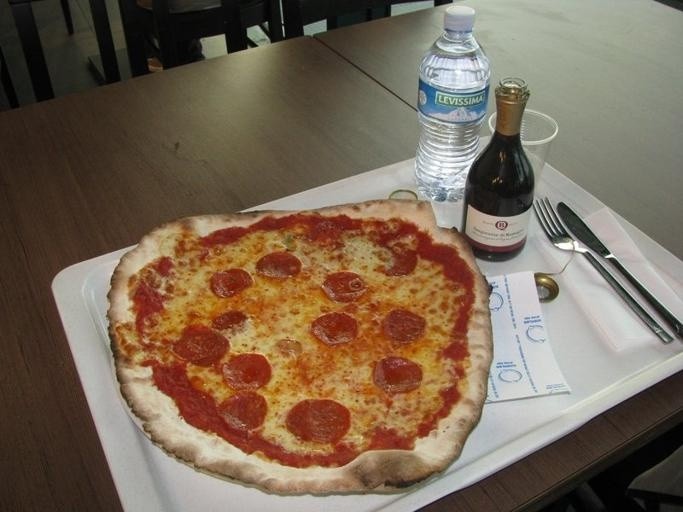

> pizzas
[106,199,495,496]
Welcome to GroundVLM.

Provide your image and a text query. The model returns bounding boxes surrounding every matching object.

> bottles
[412,5,491,207]
[454,76,536,264]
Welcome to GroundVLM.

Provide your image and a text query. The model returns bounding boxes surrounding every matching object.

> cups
[487,110,560,189]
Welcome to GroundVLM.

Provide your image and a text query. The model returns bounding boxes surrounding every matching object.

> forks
[531,197,674,344]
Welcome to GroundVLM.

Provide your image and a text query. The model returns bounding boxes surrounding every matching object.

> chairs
[116,1,284,77]
[284,1,452,46]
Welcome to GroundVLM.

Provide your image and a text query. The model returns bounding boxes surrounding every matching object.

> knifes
[556,201,683,344]
